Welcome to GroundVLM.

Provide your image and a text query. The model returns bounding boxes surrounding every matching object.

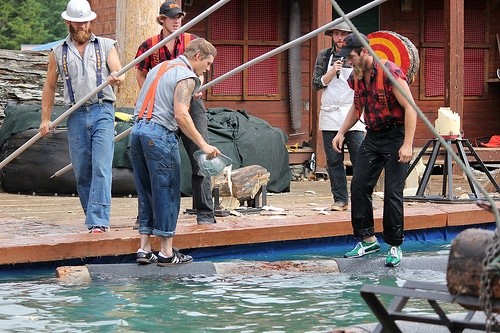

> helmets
[192,148,233,177]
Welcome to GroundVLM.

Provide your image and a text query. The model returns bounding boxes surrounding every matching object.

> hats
[60,0,98,23]
[324,17,354,36]
[159,1,184,18]
[335,33,370,57]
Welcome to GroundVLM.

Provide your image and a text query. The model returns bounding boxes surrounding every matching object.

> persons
[134,0,230,217]
[39,0,125,234]
[128,36,222,268]
[312,22,365,212]
[335,32,417,268]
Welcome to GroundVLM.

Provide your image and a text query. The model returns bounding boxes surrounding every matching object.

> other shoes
[88,225,110,235]
[330,200,349,211]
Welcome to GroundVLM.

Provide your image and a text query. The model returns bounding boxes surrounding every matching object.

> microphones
[334,53,341,78]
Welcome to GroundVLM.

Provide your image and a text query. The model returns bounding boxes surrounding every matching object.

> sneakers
[136,247,159,264]
[385,245,403,267]
[343,237,381,258]
[156,247,193,267]
